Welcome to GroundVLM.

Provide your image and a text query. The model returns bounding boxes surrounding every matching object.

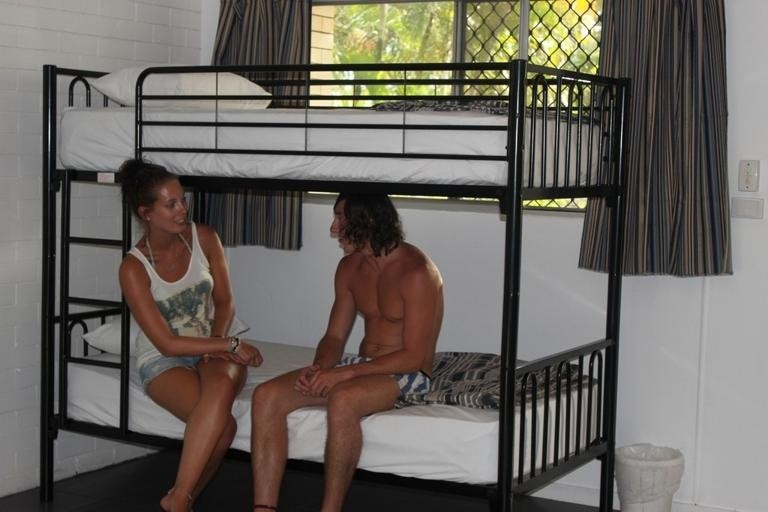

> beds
[40,62,633,512]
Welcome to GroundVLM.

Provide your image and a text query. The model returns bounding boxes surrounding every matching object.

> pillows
[83,315,249,358]
[92,67,274,108]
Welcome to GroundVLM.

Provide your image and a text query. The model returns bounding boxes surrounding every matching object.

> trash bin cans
[614,443,685,512]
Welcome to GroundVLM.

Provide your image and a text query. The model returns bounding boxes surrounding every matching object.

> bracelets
[224,336,241,354]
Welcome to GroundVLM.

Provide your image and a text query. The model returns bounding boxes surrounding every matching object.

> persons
[115,158,264,512]
[249,188,444,512]
